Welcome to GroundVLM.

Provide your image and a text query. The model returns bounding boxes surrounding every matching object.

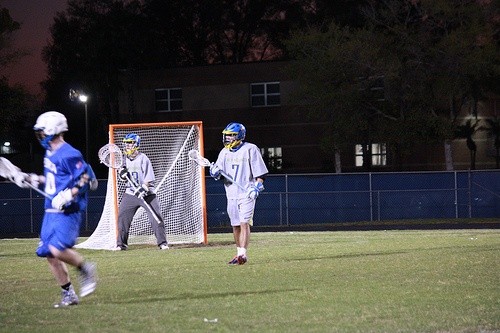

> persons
[210,123,268,265]
[15,111,98,308]
[115,133,169,250]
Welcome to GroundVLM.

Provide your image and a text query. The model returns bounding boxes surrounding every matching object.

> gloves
[118,165,130,180]
[133,184,150,198]
[209,162,223,181]
[246,181,264,198]
[9,171,40,189]
[51,189,75,211]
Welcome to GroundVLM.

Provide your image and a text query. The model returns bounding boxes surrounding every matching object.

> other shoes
[61,284,79,305]
[117,246,128,251]
[80,265,96,297]
[228,257,238,264]
[238,254,246,264]
[160,243,169,249]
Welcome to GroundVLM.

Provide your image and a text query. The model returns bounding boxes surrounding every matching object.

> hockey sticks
[0,156,65,209]
[98,143,162,226]
[187,149,247,192]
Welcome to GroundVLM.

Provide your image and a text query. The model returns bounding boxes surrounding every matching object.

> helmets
[33,111,69,150]
[123,133,140,154]
[222,123,245,149]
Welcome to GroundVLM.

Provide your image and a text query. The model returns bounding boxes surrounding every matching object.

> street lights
[68,87,90,230]
